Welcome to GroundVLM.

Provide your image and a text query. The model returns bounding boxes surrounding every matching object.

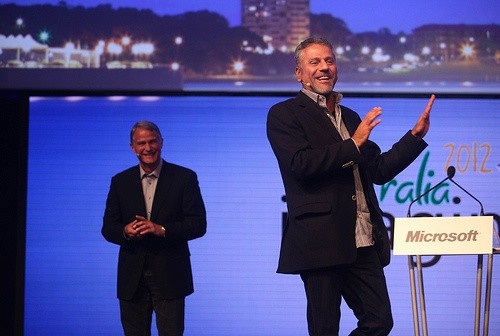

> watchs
[160,225,166,241]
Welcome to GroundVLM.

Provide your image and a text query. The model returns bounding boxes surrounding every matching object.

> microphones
[407,166,485,216]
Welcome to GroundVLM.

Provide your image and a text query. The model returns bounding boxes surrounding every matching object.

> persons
[101,121,208,336]
[267,34,438,335]
